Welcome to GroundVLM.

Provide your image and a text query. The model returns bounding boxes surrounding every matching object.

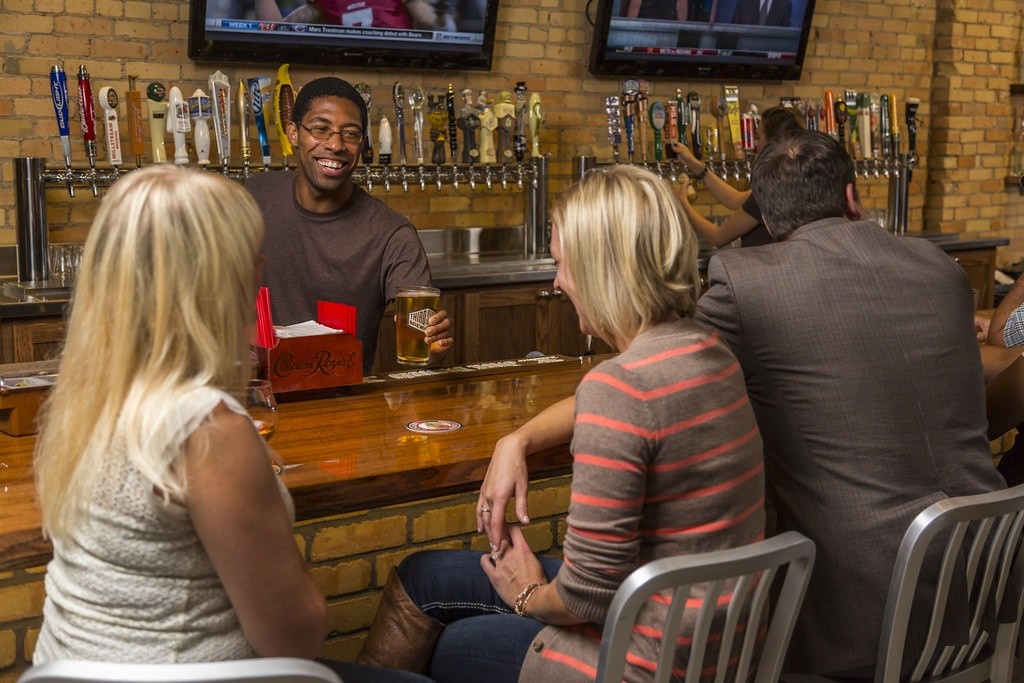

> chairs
[872,482,1024,683]
[592,526,817,682]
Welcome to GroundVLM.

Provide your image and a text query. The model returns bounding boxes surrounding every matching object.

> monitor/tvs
[186,0,497,74]
[588,1,816,82]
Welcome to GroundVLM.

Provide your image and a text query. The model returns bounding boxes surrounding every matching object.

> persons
[251,0,440,29]
[29,164,432,683]
[456,80,529,164]
[237,77,455,376]
[688,126,1021,681]
[668,108,804,248]
[356,164,771,681]
[977,268,1024,490]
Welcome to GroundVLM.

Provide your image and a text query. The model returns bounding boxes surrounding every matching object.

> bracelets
[694,164,707,179]
[512,581,553,619]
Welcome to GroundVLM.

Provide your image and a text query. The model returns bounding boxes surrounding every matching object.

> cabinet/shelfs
[933,235,1010,311]
[0,279,71,365]
[371,252,460,378]
[460,265,613,365]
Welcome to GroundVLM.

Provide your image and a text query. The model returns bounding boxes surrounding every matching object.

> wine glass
[230,378,281,475]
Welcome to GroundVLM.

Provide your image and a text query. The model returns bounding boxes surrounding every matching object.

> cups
[47,243,85,279]
[393,284,440,366]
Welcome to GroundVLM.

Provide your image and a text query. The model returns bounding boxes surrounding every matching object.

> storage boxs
[254,324,361,396]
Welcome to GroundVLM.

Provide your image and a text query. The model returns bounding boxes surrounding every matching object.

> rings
[491,552,504,563]
[481,508,491,513]
